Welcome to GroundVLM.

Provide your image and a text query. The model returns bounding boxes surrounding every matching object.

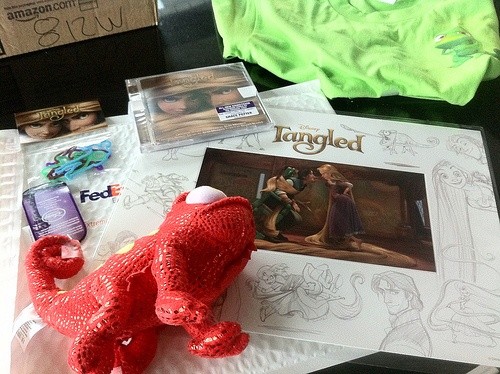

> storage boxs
[0,0,159,56]
